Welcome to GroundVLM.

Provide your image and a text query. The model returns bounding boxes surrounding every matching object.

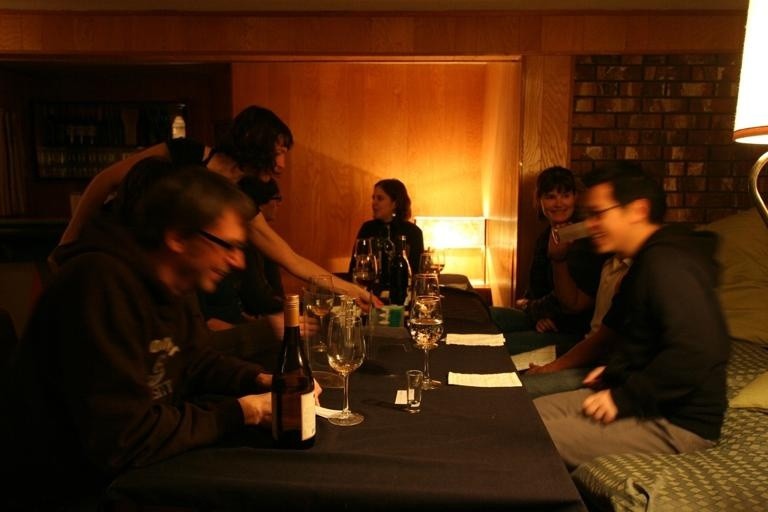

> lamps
[728,1,768,220]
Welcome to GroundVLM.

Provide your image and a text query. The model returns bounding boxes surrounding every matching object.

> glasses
[587,204,621,221]
[195,226,249,264]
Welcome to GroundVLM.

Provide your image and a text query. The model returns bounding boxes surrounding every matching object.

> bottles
[379,224,411,306]
[271,293,317,451]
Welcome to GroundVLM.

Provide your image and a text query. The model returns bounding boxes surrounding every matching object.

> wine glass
[409,295,446,392]
[306,275,335,354]
[326,316,367,429]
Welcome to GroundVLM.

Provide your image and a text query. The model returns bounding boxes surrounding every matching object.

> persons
[349,178,424,306]
[199,243,320,355]
[531,160,731,467]
[489,165,617,356]
[518,220,634,400]
[14,172,321,510]
[64,103,386,315]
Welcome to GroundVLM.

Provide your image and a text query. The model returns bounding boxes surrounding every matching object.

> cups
[405,369,424,415]
[352,238,373,266]
[413,272,442,317]
[421,248,446,273]
[353,254,379,286]
